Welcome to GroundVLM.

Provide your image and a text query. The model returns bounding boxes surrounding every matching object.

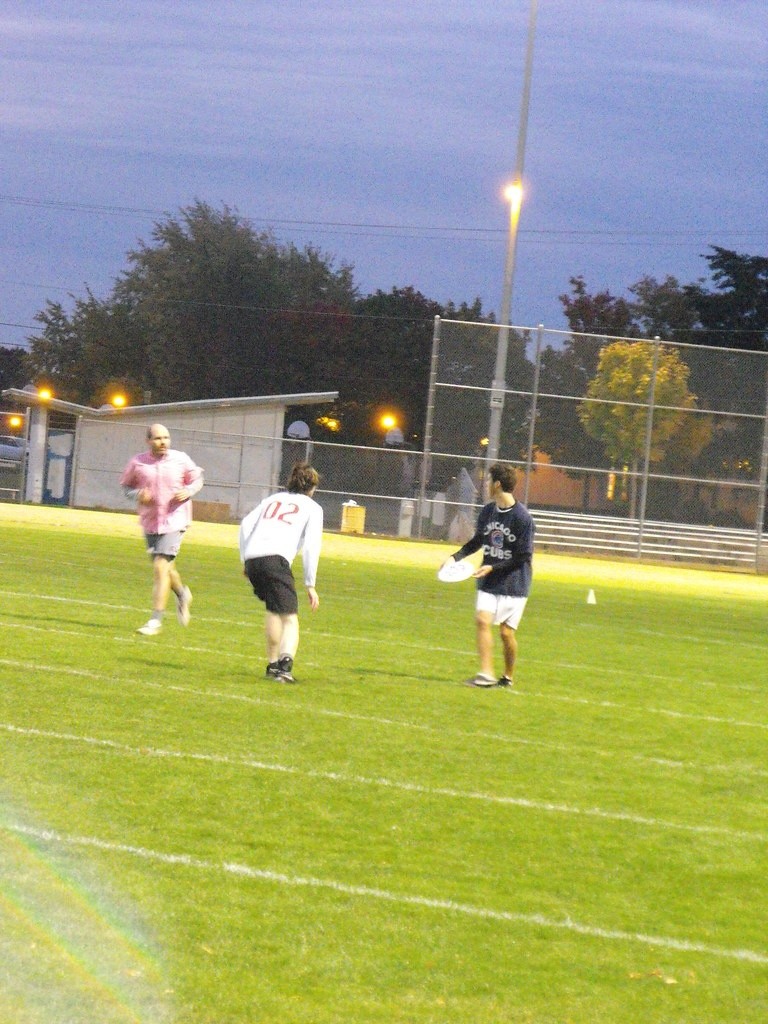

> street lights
[479,178,524,508]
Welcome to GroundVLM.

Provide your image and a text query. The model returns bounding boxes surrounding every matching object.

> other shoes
[136,619,162,635]
[178,586,192,626]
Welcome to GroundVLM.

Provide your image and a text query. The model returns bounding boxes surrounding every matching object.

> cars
[0,435,25,462]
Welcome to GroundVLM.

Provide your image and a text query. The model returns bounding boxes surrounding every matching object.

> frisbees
[436,560,476,582]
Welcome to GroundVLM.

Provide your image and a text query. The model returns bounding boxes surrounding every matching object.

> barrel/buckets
[340,506,366,534]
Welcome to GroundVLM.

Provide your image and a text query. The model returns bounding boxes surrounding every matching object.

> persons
[119,424,204,636]
[441,462,537,690]
[238,465,324,686]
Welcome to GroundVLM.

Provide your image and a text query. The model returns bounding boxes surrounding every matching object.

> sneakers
[267,664,279,678]
[498,676,513,688]
[471,672,497,687]
[279,670,295,684]
